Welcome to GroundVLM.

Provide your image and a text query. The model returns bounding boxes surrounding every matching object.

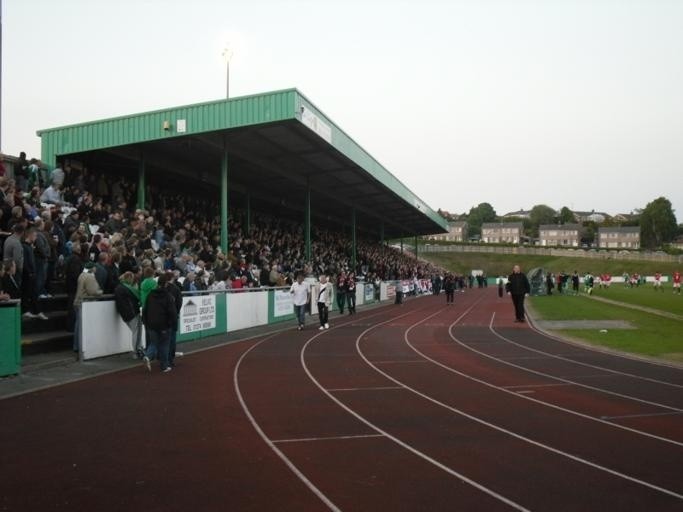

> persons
[0,151,488,376]
[497,269,682,298]
[505,265,529,322]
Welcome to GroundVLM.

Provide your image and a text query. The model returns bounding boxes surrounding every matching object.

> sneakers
[138,347,145,357]
[22,312,38,319]
[324,323,329,329]
[39,311,48,320]
[174,351,183,358]
[143,356,151,372]
[159,366,172,374]
[318,326,323,330]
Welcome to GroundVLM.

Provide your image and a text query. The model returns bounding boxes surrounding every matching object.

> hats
[84,263,97,269]
